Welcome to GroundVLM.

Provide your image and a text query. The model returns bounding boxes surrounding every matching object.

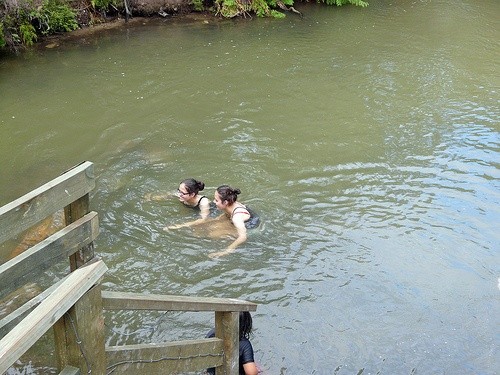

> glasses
[177,188,192,196]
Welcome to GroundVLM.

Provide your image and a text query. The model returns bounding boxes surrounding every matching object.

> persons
[146,178,264,258]
[203,312,262,375]
